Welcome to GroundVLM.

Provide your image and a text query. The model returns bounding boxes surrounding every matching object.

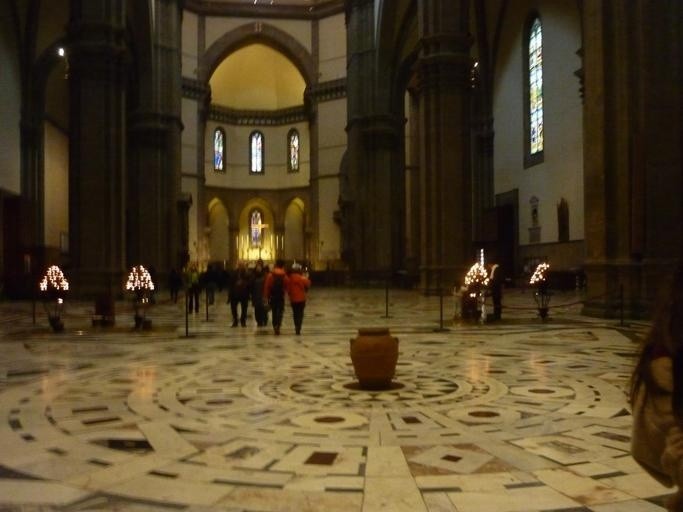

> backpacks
[268,271,288,297]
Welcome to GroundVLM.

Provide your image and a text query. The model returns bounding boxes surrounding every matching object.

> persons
[483,257,505,321]
[625,255,682,511]
[163,255,312,337]
